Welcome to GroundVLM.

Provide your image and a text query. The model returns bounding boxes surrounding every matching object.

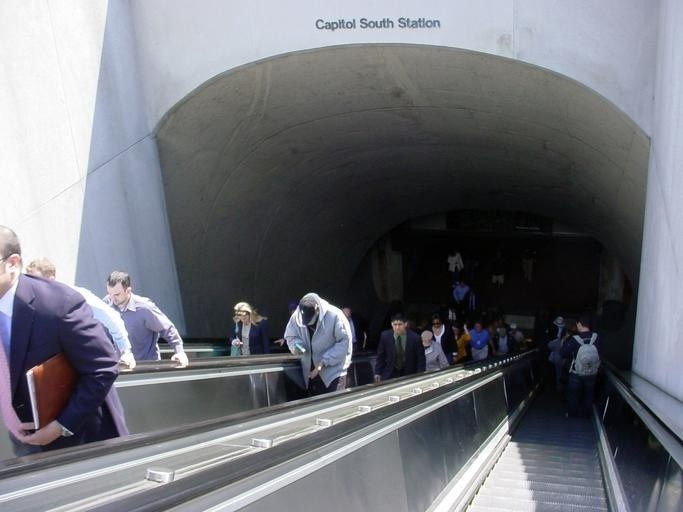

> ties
[396,335,402,371]
[0,336,26,441]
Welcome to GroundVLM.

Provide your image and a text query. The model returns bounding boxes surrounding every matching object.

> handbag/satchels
[230,345,241,356]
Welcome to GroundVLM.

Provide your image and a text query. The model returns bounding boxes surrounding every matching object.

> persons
[272,299,300,347]
[339,245,532,386]
[101,270,190,369]
[228,301,270,358]
[557,317,601,422]
[545,314,577,394]
[26,258,136,368]
[0,229,129,457]
[282,293,353,399]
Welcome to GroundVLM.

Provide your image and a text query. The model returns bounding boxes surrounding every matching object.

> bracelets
[60,427,72,437]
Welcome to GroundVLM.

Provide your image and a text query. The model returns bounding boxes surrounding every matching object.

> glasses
[432,323,439,325]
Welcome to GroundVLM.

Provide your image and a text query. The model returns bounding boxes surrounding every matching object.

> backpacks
[568,333,601,376]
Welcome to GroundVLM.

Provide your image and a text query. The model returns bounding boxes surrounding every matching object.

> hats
[420,330,432,347]
[552,316,565,327]
[299,295,318,325]
